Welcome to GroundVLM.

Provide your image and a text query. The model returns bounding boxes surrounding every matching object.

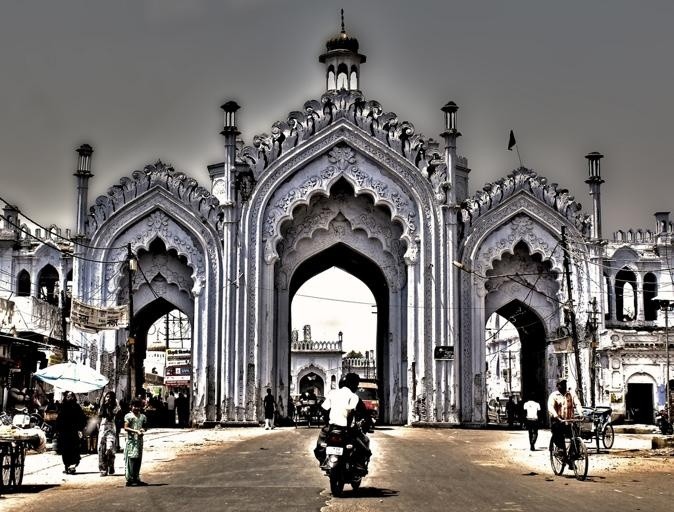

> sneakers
[126,479,149,486]
[568,465,577,470]
[101,466,115,477]
[557,448,565,458]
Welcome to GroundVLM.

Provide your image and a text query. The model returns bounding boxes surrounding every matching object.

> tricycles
[581,404,616,453]
[292,403,322,430]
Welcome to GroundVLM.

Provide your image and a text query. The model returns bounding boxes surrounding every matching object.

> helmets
[339,375,358,392]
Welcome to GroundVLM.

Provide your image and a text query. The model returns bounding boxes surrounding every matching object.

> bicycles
[547,415,590,483]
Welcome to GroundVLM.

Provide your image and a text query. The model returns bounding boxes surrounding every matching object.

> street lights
[450,258,586,408]
[652,291,674,414]
[54,238,74,357]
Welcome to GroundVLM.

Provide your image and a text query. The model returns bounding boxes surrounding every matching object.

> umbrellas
[31,361,109,394]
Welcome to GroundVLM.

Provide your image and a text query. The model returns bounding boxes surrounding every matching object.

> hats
[556,378,568,387]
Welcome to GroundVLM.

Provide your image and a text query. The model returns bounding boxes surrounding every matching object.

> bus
[355,381,380,421]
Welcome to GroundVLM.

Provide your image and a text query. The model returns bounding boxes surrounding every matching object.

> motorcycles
[315,407,379,498]
[650,404,673,436]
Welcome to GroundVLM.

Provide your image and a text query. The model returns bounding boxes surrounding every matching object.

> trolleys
[0,422,41,492]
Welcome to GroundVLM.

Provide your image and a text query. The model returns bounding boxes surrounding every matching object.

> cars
[486,397,519,425]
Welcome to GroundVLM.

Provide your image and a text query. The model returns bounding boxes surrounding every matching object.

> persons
[140,391,188,428]
[56,391,87,475]
[505,395,515,429]
[313,372,371,472]
[338,375,376,464]
[97,390,122,477]
[494,396,502,423]
[122,396,148,486]
[546,377,585,459]
[522,393,541,451]
[299,391,306,399]
[486,402,491,422]
[307,388,316,400]
[516,396,524,428]
[262,388,277,429]
